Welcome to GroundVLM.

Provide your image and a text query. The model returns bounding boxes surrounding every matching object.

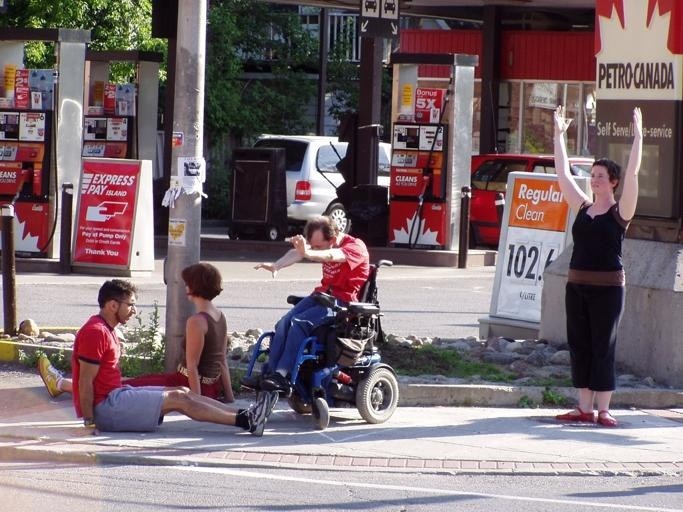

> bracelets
[84,415,96,427]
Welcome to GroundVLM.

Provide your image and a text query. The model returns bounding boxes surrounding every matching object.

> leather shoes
[555,407,593,422]
[596,411,617,427]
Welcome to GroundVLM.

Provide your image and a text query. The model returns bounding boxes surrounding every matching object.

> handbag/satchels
[334,337,368,367]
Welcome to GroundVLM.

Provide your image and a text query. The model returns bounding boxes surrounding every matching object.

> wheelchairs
[236,258,402,436]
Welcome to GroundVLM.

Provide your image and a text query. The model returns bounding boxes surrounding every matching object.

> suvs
[256,132,395,242]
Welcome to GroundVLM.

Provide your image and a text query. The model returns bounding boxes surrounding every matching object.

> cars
[469,153,597,247]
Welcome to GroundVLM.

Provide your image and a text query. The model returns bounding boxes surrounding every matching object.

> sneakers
[38,356,63,399]
[240,371,289,437]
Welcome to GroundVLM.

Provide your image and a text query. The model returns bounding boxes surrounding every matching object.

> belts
[176,364,221,385]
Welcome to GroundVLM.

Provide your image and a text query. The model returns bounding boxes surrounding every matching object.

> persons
[71,280,270,437]
[238,217,370,397]
[553,105,643,426]
[38,263,235,404]
